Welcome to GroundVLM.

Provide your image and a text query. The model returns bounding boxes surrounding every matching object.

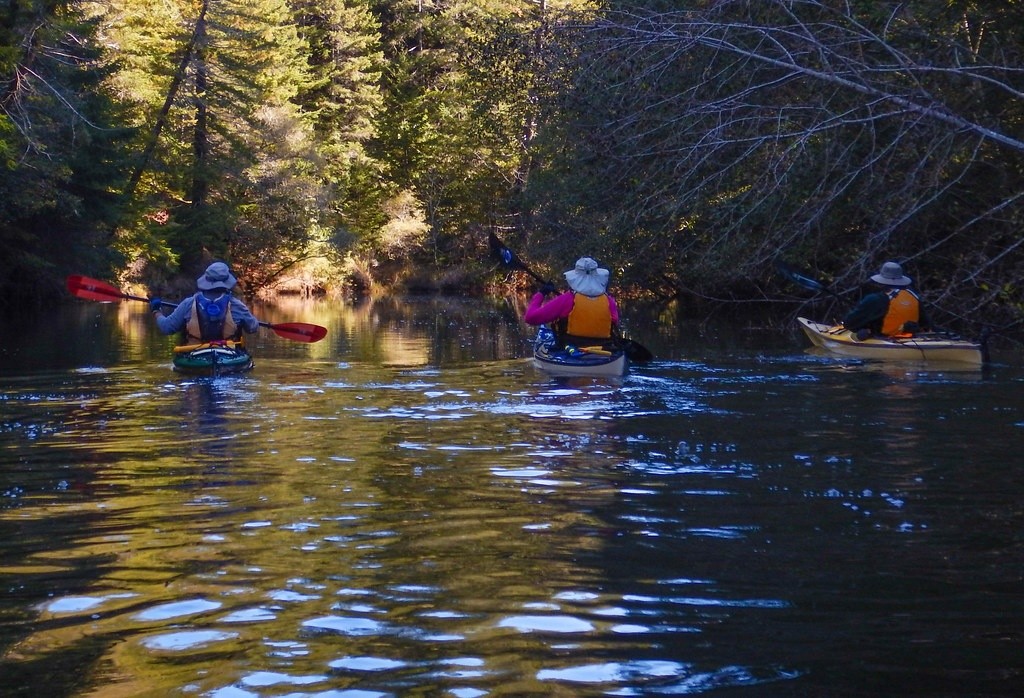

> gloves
[149,297,163,312]
[539,279,558,297]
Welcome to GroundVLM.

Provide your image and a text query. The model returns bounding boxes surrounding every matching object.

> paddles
[64,273,328,344]
[486,230,657,368]
[776,260,856,306]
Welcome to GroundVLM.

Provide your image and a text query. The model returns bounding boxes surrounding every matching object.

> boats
[533,324,631,375]
[174,338,256,376]
[798,317,983,365]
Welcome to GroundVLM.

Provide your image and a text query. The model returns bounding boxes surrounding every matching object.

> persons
[841,262,919,342]
[524,258,619,348]
[149,262,259,345]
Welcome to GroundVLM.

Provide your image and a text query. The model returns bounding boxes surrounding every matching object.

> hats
[562,254,612,297]
[870,260,912,286]
[196,260,237,292]
